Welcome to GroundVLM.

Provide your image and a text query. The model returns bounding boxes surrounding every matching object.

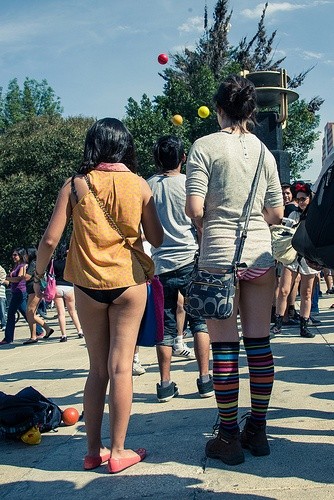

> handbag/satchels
[44,259,55,302]
[137,276,164,347]
[269,225,297,265]
[183,270,235,320]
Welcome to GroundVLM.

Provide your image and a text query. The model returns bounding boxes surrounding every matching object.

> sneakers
[156,381,178,402]
[239,412,270,456]
[132,361,146,375]
[205,415,244,465]
[173,348,191,357]
[197,374,215,397]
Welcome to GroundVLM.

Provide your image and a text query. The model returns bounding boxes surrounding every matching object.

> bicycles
[2,294,60,325]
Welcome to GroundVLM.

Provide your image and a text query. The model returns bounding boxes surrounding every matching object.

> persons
[34,118,164,472]
[185,75,285,466]
[0,245,54,345]
[45,243,84,341]
[267,181,334,338]
[139,136,215,401]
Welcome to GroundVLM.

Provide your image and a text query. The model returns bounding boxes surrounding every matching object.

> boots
[270,314,283,334]
[300,317,315,338]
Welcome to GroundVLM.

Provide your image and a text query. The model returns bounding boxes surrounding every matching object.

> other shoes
[43,329,54,338]
[108,448,145,473]
[83,448,111,469]
[282,316,298,325]
[288,305,300,320]
[307,316,322,326]
[0,338,9,344]
[78,333,84,338]
[60,337,67,342]
[23,338,38,344]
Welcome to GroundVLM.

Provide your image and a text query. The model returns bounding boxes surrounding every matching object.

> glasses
[295,197,305,204]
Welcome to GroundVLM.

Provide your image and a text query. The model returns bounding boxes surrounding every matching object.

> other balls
[62,408,80,425]
[157,54,168,65]
[198,106,210,118]
[172,115,184,126]
